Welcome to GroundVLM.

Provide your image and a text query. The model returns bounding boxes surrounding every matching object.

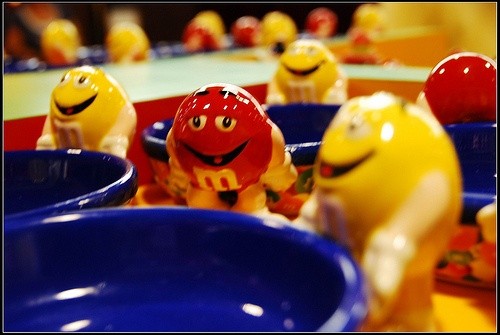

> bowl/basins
[140,102,343,206]
[4,149,138,221]
[3,206,367,332]
[435,123,496,290]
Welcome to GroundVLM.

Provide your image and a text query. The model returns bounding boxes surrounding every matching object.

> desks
[3,34,497,333]
